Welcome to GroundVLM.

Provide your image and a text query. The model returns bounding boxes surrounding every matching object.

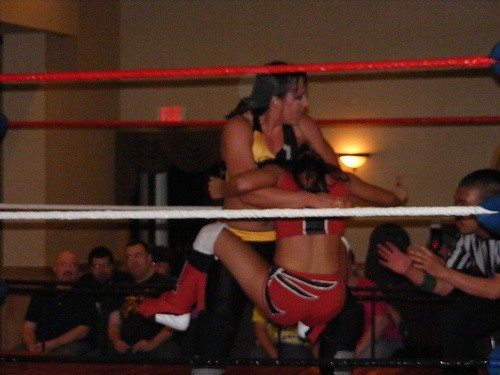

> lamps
[334,140,370,171]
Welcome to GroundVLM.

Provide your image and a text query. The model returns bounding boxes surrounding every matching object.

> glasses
[126,252,142,261]
[89,262,113,271]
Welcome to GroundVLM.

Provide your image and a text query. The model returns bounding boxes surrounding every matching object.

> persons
[250,167,500,375]
[22,241,190,365]
[191,60,365,375]
[138,138,407,326]
